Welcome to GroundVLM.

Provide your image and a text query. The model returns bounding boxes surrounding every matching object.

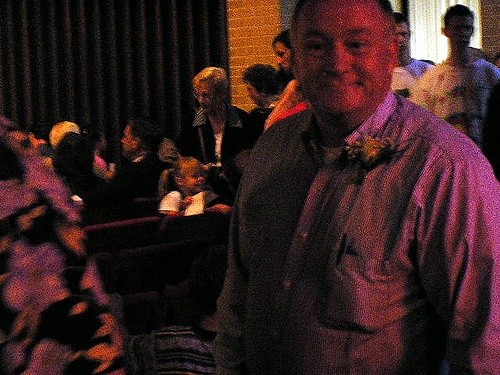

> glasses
[194,90,214,98]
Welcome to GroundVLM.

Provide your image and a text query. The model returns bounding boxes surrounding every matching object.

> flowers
[344,134,418,186]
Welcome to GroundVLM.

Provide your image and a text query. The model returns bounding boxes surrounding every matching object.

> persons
[390,4,500,178]
[115,31,297,220]
[1,117,125,375]
[212,0,500,374]
